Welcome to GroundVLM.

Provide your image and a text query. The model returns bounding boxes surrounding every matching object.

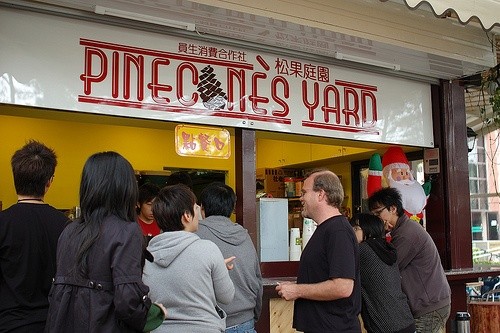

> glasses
[373,204,393,217]
[352,226,361,232]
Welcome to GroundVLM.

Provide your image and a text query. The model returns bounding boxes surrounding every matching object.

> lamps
[467,126,478,153]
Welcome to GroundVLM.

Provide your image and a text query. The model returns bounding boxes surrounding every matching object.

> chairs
[481,276,500,302]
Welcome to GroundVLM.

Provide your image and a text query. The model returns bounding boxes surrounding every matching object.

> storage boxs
[263,174,285,198]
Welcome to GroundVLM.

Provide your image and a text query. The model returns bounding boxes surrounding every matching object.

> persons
[136,171,204,237]
[276,169,362,333]
[256,182,273,202]
[367,185,451,333]
[0,139,73,333]
[45,152,167,333]
[349,212,416,333]
[193,182,263,333]
[366,146,431,226]
[142,184,237,333]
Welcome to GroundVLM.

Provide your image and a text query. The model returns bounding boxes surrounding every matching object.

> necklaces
[18,198,44,202]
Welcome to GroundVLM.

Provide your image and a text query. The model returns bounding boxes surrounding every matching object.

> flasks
[455,310,471,333]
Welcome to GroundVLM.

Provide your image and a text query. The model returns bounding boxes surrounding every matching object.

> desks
[254,267,500,333]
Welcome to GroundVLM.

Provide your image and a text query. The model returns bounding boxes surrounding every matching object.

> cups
[289,228,301,261]
[303,218,318,251]
